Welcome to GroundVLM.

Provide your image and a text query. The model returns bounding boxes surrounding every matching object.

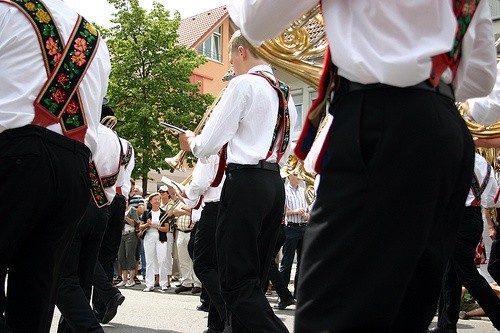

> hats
[158,186,169,192]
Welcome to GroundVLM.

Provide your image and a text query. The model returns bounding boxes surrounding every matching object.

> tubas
[240,4,328,90]
[280,154,315,205]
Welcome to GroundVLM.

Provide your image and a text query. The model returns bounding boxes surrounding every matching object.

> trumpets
[165,85,228,170]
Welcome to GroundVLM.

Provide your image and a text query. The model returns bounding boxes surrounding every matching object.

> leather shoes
[101,293,125,324]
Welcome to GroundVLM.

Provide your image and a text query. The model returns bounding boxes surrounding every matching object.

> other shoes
[274,298,295,310]
[196,305,208,312]
[266,287,272,295]
[175,286,192,292]
[112,276,182,287]
[429,327,457,333]
[192,287,202,293]
[459,311,468,319]
[161,286,168,290]
[203,328,222,333]
[144,287,154,291]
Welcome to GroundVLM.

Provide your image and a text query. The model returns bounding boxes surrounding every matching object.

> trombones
[157,174,192,227]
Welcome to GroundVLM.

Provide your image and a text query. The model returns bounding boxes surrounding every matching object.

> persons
[90,0,500,333]
[0,0,112,333]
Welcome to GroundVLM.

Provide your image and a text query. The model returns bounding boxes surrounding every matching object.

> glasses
[159,192,166,193]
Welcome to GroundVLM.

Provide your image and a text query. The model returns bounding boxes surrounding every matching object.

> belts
[225,160,279,172]
[177,229,192,233]
[115,195,126,199]
[288,222,307,226]
[333,78,456,101]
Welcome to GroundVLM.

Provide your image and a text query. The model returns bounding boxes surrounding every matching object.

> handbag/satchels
[138,210,149,239]
